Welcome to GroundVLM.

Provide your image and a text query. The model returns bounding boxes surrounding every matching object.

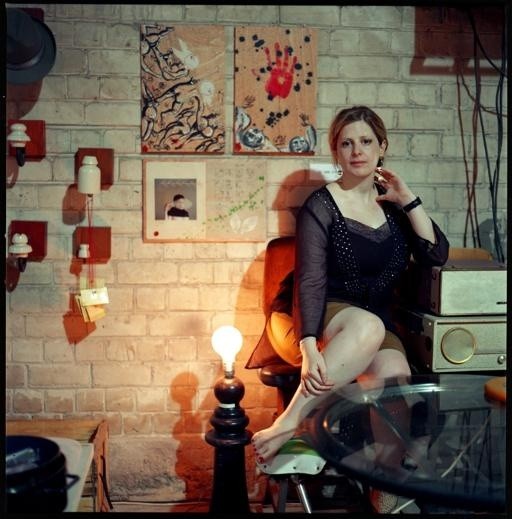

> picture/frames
[145,161,207,240]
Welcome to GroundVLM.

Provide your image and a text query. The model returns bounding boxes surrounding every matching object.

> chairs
[257,234,303,415]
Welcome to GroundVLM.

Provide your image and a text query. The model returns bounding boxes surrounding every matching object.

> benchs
[4,418,109,512]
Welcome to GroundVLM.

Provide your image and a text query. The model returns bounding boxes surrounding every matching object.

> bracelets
[400,193,423,213]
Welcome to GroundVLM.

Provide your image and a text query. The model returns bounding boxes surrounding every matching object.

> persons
[249,105,449,513]
[164,190,194,220]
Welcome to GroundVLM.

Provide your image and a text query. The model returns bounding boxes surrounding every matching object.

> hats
[6,8,56,86]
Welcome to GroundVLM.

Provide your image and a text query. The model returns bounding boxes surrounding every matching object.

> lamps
[4,117,114,272]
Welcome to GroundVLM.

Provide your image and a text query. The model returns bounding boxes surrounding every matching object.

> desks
[298,369,508,516]
